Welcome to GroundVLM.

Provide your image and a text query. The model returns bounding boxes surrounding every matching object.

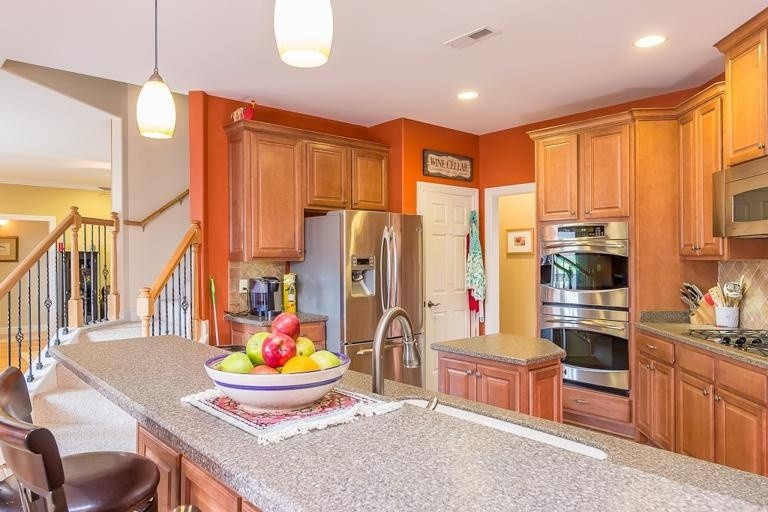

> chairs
[2,366,160,512]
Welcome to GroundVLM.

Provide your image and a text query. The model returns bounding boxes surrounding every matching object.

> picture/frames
[505,228,534,258]
[0,236,19,262]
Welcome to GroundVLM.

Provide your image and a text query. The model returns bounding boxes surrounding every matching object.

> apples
[219,312,340,374]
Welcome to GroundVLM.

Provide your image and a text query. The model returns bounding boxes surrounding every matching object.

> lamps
[135,0,177,140]
[273,2,334,68]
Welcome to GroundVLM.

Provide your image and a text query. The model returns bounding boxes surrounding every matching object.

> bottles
[288,286,294,302]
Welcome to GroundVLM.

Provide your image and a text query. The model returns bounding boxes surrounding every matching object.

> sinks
[397,397,610,462]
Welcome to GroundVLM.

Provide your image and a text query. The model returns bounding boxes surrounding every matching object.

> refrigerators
[290,207,426,388]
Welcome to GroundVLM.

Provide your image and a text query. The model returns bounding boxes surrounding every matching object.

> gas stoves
[685,327,768,358]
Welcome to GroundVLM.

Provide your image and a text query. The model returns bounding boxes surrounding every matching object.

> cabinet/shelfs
[431,333,567,424]
[633,332,675,454]
[675,97,724,258]
[304,129,392,213]
[180,455,263,512]
[674,343,768,476]
[537,123,633,223]
[725,26,768,165]
[222,120,304,263]
[134,421,182,512]
[228,310,330,355]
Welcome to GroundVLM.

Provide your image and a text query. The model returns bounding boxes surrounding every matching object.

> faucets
[370,305,420,394]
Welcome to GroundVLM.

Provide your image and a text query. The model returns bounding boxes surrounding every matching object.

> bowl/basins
[206,349,351,413]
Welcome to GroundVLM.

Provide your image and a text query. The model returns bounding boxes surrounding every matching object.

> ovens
[537,221,631,395]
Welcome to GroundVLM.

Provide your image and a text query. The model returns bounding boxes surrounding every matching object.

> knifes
[680,282,704,309]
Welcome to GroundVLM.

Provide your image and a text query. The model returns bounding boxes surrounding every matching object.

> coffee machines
[249,277,283,317]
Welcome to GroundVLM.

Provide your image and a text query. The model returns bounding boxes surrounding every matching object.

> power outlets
[239,279,248,294]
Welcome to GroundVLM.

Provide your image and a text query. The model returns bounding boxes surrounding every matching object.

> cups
[715,307,740,327]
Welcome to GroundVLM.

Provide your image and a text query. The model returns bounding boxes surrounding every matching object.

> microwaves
[710,154,768,240]
